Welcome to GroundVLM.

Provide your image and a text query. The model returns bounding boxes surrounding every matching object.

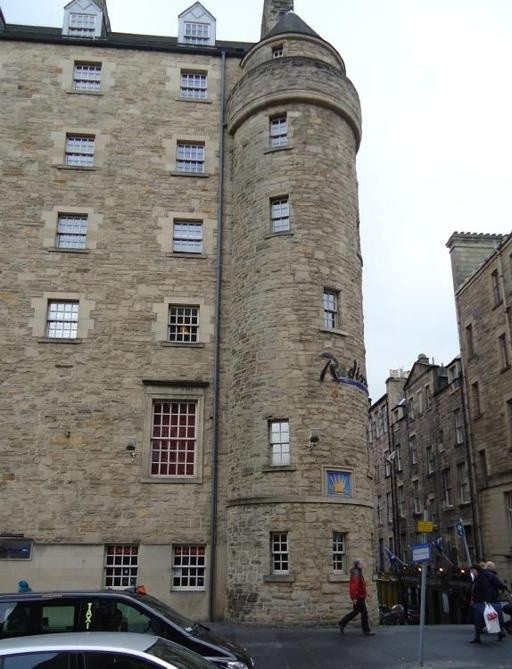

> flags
[431,537,443,553]
[386,549,400,573]
[454,520,464,539]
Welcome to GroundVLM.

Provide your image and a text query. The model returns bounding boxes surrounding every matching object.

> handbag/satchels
[483,601,502,634]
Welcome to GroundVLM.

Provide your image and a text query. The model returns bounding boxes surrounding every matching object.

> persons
[468,561,495,646]
[336,557,374,636]
[483,561,510,640]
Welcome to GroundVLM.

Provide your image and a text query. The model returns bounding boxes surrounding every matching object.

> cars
[0,590,255,669]
[379,604,430,625]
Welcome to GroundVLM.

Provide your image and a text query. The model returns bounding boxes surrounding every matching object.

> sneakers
[339,622,377,636]
[469,632,506,644]
